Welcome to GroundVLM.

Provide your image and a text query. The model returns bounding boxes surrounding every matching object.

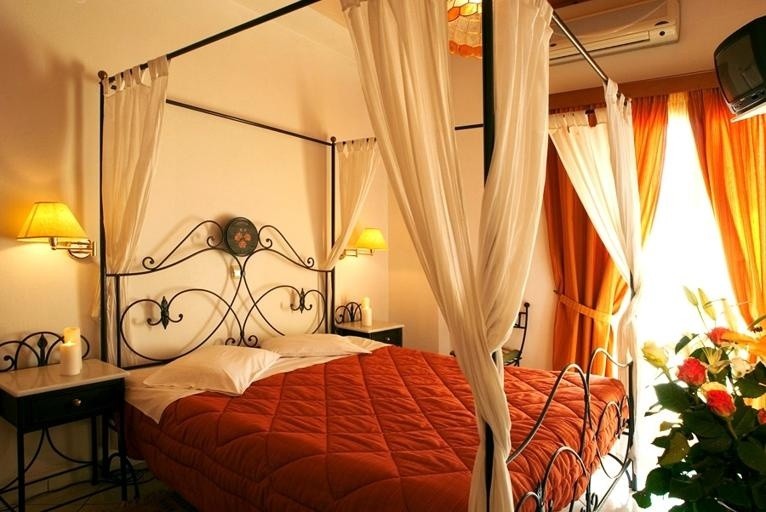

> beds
[98,0,643,512]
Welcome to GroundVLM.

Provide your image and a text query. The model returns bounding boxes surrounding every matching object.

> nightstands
[335,302,404,348]
[0,330,130,512]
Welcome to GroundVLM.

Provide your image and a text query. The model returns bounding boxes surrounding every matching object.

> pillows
[143,344,280,396]
[261,333,372,357]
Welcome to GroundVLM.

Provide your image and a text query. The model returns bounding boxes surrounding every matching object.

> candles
[61,342,78,375]
[64,326,82,370]
[360,299,369,312]
[362,307,371,326]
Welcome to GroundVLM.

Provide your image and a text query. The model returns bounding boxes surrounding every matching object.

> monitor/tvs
[713,15,766,116]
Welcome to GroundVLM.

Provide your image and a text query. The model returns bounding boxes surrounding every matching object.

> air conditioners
[549,0,681,64]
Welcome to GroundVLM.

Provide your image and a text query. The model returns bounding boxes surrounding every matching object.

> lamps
[446,0,484,60]
[338,227,390,261]
[18,202,96,260]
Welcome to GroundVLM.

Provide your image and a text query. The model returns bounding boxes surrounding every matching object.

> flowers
[630,280,766,512]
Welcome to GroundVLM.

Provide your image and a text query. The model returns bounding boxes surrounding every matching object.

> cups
[359,296,374,327]
[58,325,83,376]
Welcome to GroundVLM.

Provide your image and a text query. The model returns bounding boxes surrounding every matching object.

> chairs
[449,302,530,366]
